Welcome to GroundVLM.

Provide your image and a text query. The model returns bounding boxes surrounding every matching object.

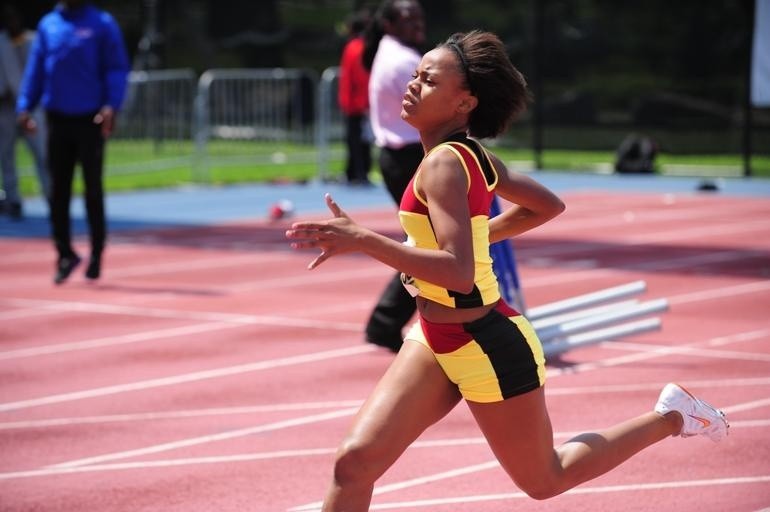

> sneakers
[55,253,79,284]
[366,329,403,353]
[2,199,20,219]
[85,251,102,279]
[655,382,730,441]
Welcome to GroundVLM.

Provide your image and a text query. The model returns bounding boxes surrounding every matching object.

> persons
[366,2,433,357]
[285,28,732,511]
[339,10,378,193]
[0,0,57,225]
[13,0,131,287]
